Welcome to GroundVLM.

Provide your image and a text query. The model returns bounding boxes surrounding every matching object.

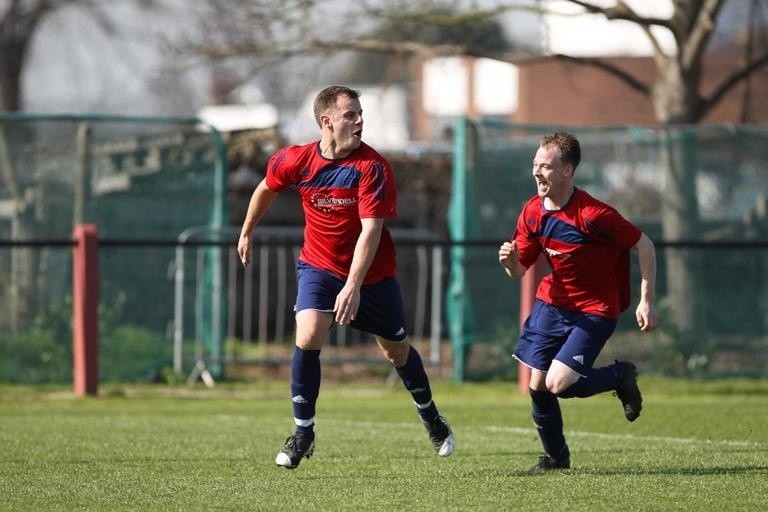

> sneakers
[528,443,570,472]
[417,410,455,457]
[608,358,642,424]
[274,431,315,470]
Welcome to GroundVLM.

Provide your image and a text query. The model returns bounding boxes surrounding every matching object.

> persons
[496,129,661,476]
[235,86,457,470]
